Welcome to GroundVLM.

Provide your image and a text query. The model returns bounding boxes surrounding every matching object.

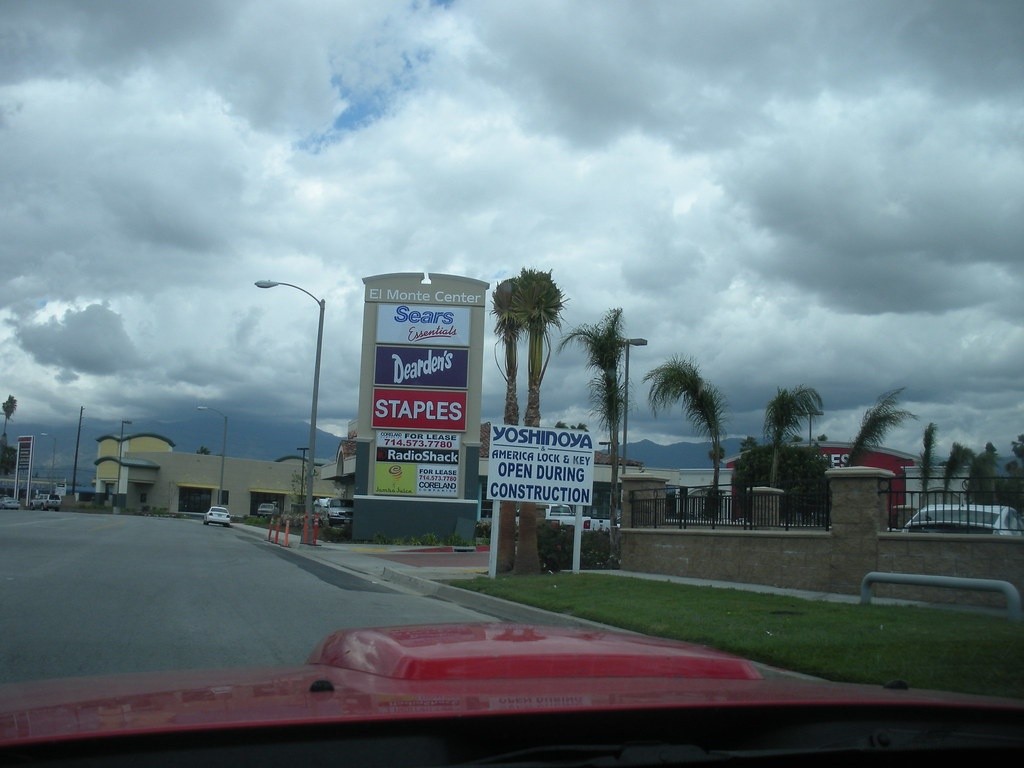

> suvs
[313,498,353,525]
[30,493,63,511]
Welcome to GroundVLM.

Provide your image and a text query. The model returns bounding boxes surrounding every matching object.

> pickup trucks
[514,503,614,531]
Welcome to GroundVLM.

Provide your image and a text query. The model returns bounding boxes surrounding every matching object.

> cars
[204,506,231,527]
[257,503,279,517]
[901,502,1024,536]
[0,496,21,510]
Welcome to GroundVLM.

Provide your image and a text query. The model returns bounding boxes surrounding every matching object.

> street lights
[255,279,327,540]
[796,409,824,446]
[599,440,621,454]
[617,336,648,472]
[40,433,56,496]
[196,405,229,507]
[295,447,311,523]
[115,419,133,507]
